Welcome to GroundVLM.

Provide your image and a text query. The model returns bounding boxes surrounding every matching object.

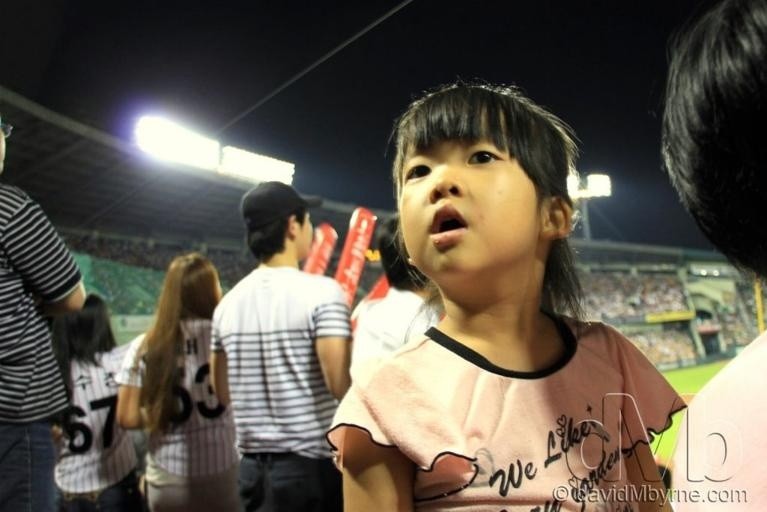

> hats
[237,180,324,232]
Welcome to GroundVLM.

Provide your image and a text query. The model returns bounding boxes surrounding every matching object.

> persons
[116,250,241,511]
[0,106,89,508]
[322,81,691,510]
[44,292,152,511]
[208,180,354,511]
[547,263,765,372]
[343,212,448,389]
[657,0,766,512]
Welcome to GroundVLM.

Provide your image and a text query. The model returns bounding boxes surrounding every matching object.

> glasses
[0,123,14,139]
